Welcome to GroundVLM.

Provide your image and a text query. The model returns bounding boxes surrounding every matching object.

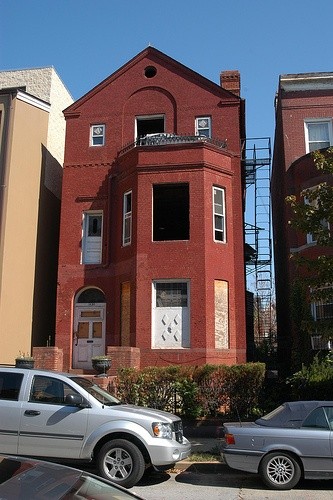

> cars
[219,401,333,491]
[1,454,152,500]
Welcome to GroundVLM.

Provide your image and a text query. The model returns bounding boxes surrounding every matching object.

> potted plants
[91,353,112,378]
[15,350,36,370]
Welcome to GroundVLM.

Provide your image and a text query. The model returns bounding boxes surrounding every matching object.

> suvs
[0,368,193,490]
